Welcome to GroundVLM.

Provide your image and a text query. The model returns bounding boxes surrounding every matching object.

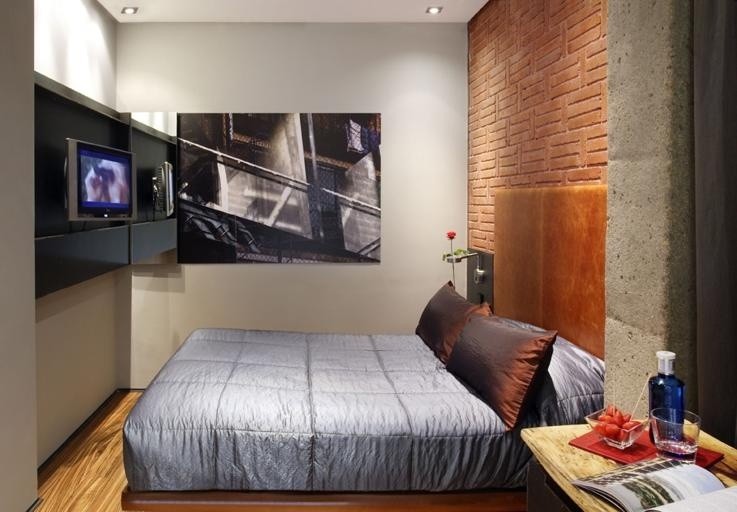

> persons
[83,159,128,205]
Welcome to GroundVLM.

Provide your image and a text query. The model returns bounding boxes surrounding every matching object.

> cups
[650,407,702,464]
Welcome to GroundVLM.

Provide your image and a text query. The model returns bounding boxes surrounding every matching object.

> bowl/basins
[583,401,648,450]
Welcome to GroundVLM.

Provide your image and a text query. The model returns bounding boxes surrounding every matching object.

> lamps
[445,252,485,285]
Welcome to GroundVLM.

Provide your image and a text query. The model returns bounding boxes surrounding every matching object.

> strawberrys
[595,405,643,442]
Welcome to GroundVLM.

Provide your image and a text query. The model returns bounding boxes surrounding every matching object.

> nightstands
[515,419,737,512]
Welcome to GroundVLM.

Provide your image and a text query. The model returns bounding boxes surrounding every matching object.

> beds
[118,184,607,512]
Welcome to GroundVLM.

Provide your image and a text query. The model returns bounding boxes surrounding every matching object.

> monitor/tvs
[66,138,137,221]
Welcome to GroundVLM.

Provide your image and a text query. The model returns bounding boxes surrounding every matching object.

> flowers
[445,231,458,290]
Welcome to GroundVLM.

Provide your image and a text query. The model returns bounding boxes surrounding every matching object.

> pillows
[411,279,561,432]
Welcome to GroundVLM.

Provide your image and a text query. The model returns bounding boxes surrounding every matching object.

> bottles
[648,350,685,446]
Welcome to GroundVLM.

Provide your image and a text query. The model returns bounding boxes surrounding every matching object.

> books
[569,455,737,512]
